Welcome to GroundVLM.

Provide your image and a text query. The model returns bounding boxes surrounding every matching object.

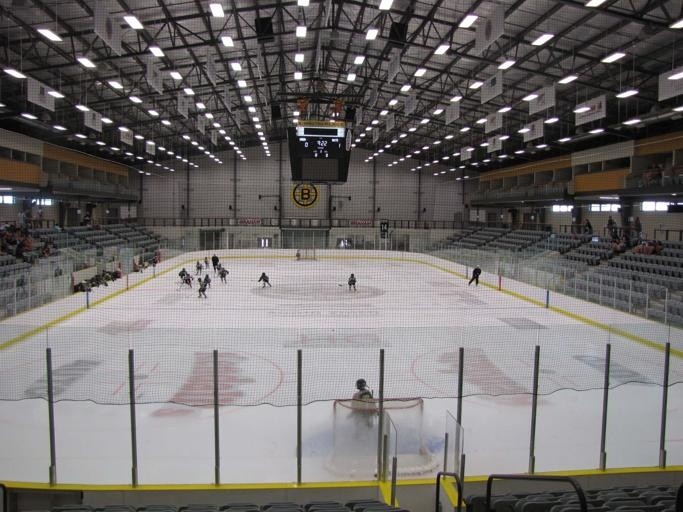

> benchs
[0,223,171,321]
[428,227,683,327]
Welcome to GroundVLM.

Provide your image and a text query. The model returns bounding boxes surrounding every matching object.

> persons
[345,377,377,438]
[347,273,357,294]
[15,274,26,287]
[53,265,63,278]
[607,216,616,236]
[70,248,229,299]
[81,211,91,227]
[0,203,54,267]
[466,264,482,285]
[257,271,272,290]
[585,219,593,235]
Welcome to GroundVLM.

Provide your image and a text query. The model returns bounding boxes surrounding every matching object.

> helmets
[356,379,368,389]
